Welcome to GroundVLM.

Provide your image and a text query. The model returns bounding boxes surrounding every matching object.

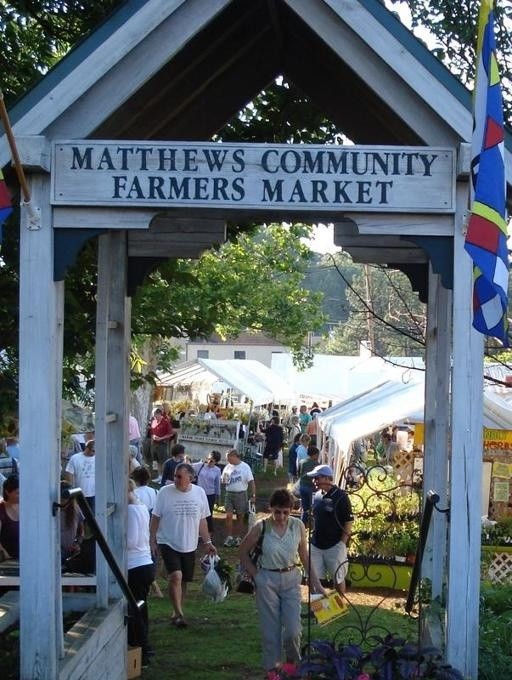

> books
[310,589,350,627]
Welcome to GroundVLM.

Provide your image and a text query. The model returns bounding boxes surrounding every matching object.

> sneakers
[146,593,164,600]
[265,671,280,680]
[169,614,177,624]
[298,610,316,619]
[234,537,242,547]
[223,536,234,547]
[282,663,297,674]
[176,615,187,628]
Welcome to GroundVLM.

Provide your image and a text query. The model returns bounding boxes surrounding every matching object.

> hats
[306,464,333,479]
[292,406,298,411]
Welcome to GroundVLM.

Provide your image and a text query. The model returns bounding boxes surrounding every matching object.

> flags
[461,0,512,350]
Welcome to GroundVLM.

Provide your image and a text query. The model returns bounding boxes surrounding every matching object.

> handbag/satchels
[233,519,266,596]
[241,500,257,528]
[192,462,205,485]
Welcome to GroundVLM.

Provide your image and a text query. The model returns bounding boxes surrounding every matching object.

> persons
[298,405,311,433]
[61,481,86,566]
[305,411,319,446]
[129,444,141,479]
[292,446,321,529]
[300,464,352,619]
[129,415,141,465]
[150,408,174,485]
[284,405,298,428]
[220,448,257,548]
[370,426,416,469]
[149,463,218,627]
[185,450,221,544]
[0,476,19,563]
[131,466,164,598]
[161,444,185,487]
[295,432,312,480]
[282,416,301,450]
[65,440,95,535]
[238,488,336,680]
[288,432,303,485]
[259,417,284,476]
[128,479,156,669]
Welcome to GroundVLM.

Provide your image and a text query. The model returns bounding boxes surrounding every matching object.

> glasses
[88,447,95,453]
[206,457,215,463]
[271,508,292,516]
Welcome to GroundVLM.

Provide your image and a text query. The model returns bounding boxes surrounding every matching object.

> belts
[257,562,304,574]
